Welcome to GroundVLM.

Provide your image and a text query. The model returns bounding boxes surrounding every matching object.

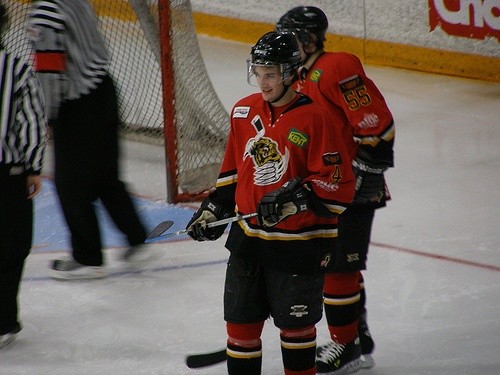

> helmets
[275,5,329,42]
[249,31,302,65]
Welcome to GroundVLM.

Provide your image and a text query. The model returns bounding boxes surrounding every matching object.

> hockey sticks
[185,346,228,369]
[143,205,283,245]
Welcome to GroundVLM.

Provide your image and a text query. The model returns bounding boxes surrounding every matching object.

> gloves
[184,198,236,241]
[255,177,305,227]
[351,162,385,208]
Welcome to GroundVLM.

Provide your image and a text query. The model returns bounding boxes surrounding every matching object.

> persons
[186,31,355,375]
[0,1,49,343]
[276,7,396,374]
[27,0,149,279]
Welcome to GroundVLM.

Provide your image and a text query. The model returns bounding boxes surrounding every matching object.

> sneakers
[0,324,22,348]
[314,335,362,375]
[44,257,107,281]
[358,322,376,370]
[109,243,161,271]
